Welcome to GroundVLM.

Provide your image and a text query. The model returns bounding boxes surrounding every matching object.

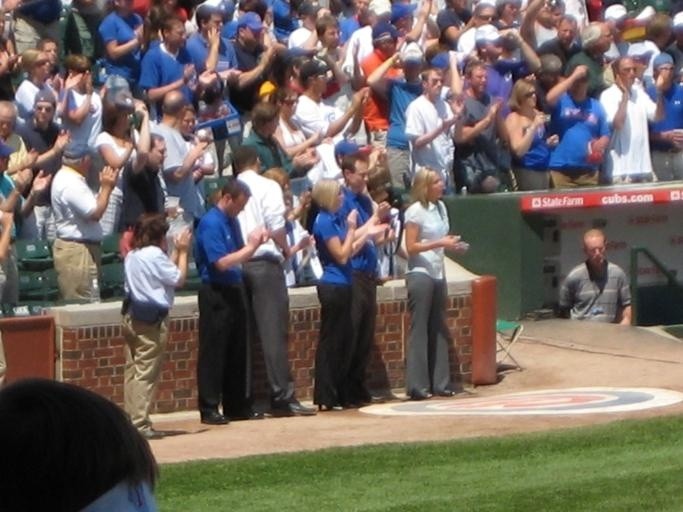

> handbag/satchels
[134,303,158,321]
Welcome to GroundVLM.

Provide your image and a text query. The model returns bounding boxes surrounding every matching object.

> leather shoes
[200,404,316,424]
[319,394,383,410]
[420,391,455,398]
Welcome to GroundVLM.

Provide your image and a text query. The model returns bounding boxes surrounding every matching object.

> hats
[674,12,683,26]
[653,54,673,67]
[605,5,627,19]
[1,88,135,159]
[335,139,372,156]
[628,42,653,57]
[219,0,504,79]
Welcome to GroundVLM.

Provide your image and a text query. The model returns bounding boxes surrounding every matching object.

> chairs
[496,319,523,374]
[0,175,236,315]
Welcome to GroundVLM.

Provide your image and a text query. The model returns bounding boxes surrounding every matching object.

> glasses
[526,92,539,97]
[284,100,298,105]
[35,60,51,66]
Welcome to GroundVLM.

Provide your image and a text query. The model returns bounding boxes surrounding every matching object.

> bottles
[88,263,100,305]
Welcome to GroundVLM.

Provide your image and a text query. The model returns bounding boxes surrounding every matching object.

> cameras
[497,35,519,51]
[542,114,552,123]
[384,182,403,208]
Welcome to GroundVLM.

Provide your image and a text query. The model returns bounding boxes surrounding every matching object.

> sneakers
[137,427,165,438]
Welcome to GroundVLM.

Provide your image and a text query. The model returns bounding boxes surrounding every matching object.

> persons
[1,0,682,439]
[1,376,163,511]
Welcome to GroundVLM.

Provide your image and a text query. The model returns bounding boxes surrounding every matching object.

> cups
[167,196,180,218]
